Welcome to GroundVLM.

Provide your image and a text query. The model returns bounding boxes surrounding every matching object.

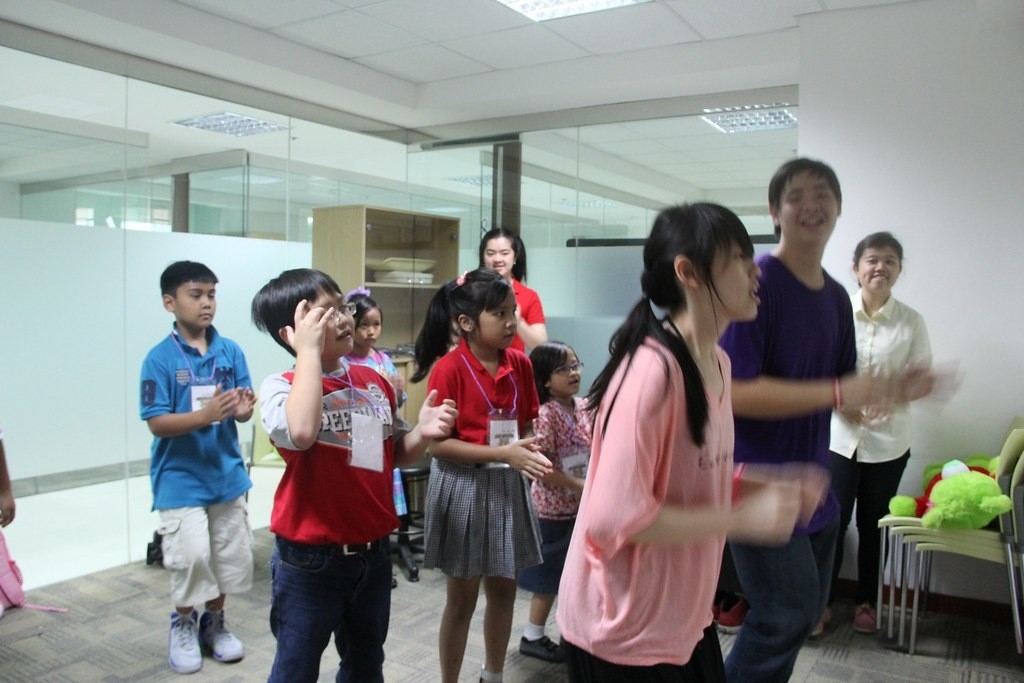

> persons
[410,266,555,683]
[717,159,937,683]
[341,288,407,515]
[249,269,458,683]
[518,341,597,662]
[0,436,15,527]
[555,203,829,683]
[479,228,548,352]
[812,231,932,635]
[140,262,258,673]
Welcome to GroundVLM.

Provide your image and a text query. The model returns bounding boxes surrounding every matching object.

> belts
[327,542,380,555]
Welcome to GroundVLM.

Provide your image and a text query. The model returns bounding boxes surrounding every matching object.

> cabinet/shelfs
[311,204,460,428]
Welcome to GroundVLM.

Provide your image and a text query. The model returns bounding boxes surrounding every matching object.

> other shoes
[812,609,833,634]
[854,605,881,633]
[717,596,751,633]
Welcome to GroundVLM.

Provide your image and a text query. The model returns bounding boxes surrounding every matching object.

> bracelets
[833,377,842,410]
[733,462,745,491]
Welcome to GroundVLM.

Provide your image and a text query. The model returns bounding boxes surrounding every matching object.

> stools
[389,462,431,581]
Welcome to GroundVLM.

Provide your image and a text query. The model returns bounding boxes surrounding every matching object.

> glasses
[324,301,356,319]
[550,362,583,377]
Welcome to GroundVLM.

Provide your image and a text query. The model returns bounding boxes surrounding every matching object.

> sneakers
[519,636,566,662]
[198,609,243,661]
[169,609,203,673]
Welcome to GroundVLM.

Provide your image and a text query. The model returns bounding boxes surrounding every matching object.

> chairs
[876,417,1024,657]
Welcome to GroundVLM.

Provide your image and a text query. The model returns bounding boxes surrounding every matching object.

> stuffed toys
[889,454,1012,531]
